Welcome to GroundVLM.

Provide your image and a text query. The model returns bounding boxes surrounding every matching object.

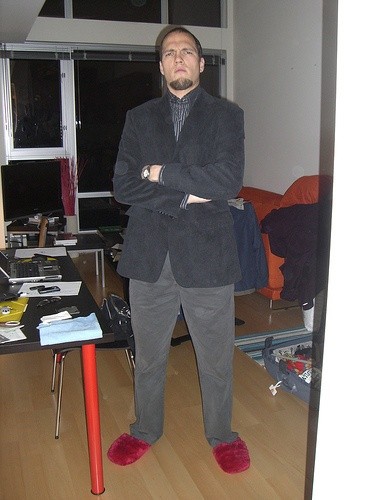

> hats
[155,25,186,62]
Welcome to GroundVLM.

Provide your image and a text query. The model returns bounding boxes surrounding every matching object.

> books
[54,236,78,246]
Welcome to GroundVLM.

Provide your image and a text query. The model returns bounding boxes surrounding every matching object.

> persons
[106,23,250,474]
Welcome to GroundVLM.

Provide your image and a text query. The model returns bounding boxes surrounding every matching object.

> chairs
[178,198,269,319]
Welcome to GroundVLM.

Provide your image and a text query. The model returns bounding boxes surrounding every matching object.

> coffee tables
[95,224,245,346]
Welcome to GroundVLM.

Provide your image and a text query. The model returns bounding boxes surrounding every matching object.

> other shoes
[107,432,152,466]
[212,436,250,474]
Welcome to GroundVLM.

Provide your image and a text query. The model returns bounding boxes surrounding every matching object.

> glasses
[36,296,62,308]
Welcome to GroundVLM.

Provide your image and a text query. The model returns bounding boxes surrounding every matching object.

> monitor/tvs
[1,160,63,225]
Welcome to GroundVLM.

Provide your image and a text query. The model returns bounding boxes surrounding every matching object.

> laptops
[0,251,62,284]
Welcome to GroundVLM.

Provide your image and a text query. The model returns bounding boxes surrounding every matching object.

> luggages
[262,335,313,403]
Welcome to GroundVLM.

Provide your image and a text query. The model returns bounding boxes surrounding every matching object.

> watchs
[143,165,152,180]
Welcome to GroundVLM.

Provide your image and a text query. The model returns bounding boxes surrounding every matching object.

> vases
[63,214,79,258]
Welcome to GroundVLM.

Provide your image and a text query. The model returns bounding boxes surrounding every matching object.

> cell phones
[38,286,60,294]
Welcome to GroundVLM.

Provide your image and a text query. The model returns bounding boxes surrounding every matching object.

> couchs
[236,174,333,309]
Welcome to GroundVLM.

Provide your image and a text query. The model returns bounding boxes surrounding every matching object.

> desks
[0,216,130,495]
